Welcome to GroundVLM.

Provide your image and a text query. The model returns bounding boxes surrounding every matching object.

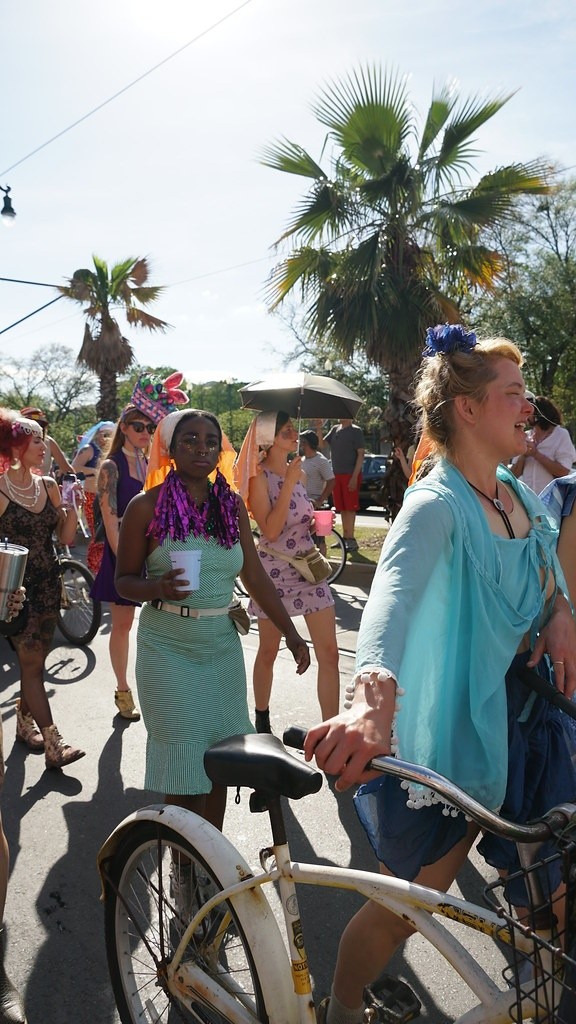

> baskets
[479,835,576,1024]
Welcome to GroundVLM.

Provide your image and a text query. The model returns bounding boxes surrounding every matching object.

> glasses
[124,420,157,435]
[96,433,114,438]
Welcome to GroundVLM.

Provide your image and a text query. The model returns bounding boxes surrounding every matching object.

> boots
[40,726,86,770]
[14,698,45,749]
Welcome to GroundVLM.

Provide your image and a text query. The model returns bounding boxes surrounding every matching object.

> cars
[326,454,390,513]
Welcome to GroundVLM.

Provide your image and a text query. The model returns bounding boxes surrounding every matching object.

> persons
[0,372,188,769]
[0,706,27,1024]
[234,411,340,733]
[114,408,310,931]
[303,322,575,1024]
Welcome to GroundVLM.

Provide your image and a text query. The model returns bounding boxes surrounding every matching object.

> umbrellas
[237,371,363,457]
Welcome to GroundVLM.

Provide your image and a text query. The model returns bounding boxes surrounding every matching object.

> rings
[554,661,563,664]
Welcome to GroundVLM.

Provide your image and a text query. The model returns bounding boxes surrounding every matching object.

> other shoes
[346,538,357,552]
[168,862,212,937]
[330,537,345,548]
[195,861,229,913]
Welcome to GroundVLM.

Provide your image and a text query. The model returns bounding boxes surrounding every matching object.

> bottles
[63,470,77,508]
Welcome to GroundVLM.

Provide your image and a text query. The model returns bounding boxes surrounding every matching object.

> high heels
[114,686,140,719]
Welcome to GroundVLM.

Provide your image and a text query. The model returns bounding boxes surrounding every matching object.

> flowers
[419,321,479,358]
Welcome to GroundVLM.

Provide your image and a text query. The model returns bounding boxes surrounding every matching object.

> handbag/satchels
[93,493,108,544]
[258,542,333,584]
[150,591,251,636]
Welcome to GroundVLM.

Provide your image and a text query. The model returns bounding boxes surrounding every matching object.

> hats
[299,429,319,450]
[20,407,48,425]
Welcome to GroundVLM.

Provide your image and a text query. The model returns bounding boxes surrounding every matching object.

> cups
[313,510,333,536]
[0,541,29,620]
[171,549,202,590]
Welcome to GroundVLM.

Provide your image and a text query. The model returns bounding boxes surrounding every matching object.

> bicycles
[97,664,575,1024]
[234,501,347,597]
[45,472,102,646]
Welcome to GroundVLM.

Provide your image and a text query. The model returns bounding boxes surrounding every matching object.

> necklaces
[467,479,516,539]
[4,470,40,508]
[121,446,143,457]
